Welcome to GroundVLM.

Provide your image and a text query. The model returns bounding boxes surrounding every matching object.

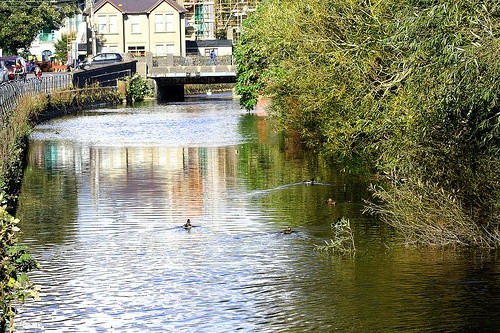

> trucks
[35,56,69,72]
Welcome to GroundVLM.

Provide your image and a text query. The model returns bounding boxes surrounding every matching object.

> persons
[210,49,217,66]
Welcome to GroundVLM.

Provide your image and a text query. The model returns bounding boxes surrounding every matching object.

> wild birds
[327,198,337,205]
[183,219,192,229]
[284,226,292,234]
[303,179,316,185]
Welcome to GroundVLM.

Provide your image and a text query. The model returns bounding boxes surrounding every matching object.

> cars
[0,56,26,80]
[79,53,124,70]
